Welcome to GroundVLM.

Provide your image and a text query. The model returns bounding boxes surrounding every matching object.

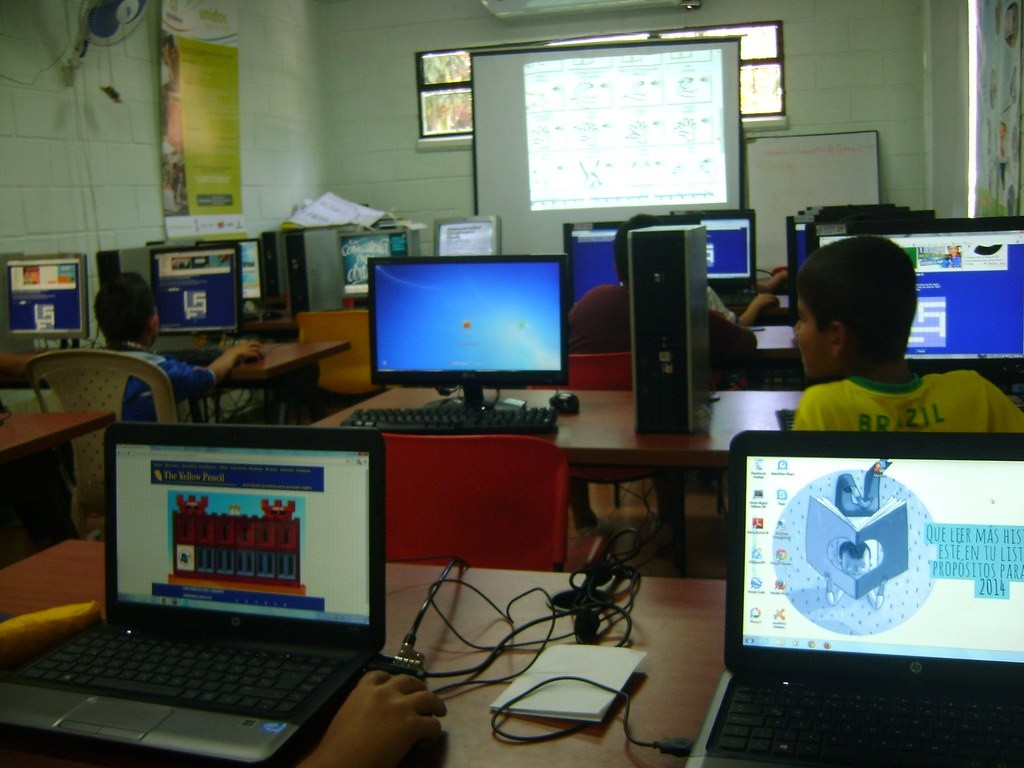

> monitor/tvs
[670,208,759,293]
[562,221,625,312]
[785,204,1024,402]
[3,215,569,413]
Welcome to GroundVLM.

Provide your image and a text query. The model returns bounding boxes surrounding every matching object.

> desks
[0,540,727,768]
[241,284,368,309]
[0,410,118,467]
[0,341,351,428]
[304,387,805,577]
[728,325,800,390]
[732,295,789,315]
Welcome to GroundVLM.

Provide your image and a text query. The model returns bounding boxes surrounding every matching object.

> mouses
[549,392,579,415]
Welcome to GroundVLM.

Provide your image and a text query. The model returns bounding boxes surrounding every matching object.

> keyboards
[338,406,557,434]
[775,409,796,430]
[159,349,239,368]
[720,292,757,306]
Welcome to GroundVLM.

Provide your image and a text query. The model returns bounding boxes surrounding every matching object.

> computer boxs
[628,224,711,433]
[96,245,181,290]
[284,226,345,317]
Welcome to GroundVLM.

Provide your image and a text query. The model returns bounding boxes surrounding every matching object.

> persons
[792,234,1024,432]
[160,34,191,216]
[294,670,447,768]
[707,283,780,329]
[756,269,790,295]
[565,213,758,544]
[57,271,264,491]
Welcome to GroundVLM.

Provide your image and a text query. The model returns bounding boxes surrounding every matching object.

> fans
[62,0,150,85]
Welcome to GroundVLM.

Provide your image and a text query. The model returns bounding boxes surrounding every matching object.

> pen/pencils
[752,328,766,331]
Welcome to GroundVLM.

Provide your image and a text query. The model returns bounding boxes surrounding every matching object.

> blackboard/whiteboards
[745,129,878,280]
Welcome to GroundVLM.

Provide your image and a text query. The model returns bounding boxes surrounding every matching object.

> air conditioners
[481,0,695,19]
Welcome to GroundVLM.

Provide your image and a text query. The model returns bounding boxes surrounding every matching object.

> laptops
[0,422,389,768]
[684,430,1024,768]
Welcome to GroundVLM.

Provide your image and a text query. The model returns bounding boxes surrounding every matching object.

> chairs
[525,351,725,569]
[296,308,380,394]
[22,348,178,540]
[375,431,606,577]
